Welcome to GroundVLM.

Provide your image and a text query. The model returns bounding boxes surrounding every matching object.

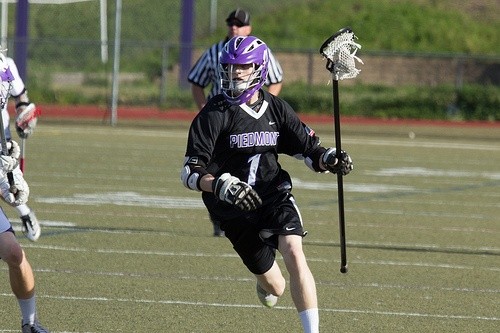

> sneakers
[214,224,225,236]
[257,281,277,308]
[20,210,41,242]
[22,319,48,333]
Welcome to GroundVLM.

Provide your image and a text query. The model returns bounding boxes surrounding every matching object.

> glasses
[227,21,249,27]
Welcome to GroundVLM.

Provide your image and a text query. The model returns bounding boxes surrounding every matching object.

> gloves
[0,140,21,172]
[214,173,262,211]
[322,148,354,175]
[15,113,37,137]
[0,166,30,207]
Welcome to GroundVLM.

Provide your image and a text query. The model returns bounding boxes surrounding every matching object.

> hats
[226,8,250,24]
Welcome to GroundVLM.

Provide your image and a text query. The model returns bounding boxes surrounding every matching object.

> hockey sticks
[0,101,17,197]
[319,28,364,274]
[20,123,26,172]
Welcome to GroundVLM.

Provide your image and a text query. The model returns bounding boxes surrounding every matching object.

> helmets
[218,36,269,104]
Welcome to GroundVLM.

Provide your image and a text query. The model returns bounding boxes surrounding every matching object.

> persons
[187,8,284,112]
[0,140,51,333]
[0,45,42,242]
[180,36,354,333]
